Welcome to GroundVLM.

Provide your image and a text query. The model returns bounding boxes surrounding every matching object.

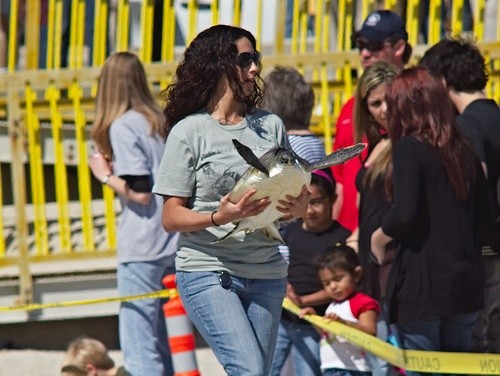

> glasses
[356,39,393,52]
[235,52,260,68]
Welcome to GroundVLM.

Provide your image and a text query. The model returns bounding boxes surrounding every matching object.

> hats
[351,9,406,41]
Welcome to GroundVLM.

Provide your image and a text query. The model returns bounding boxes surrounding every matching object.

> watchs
[102,174,113,184]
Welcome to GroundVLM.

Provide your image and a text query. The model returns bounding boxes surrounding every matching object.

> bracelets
[212,211,221,226]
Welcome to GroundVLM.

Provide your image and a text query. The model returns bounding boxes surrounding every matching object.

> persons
[66,338,134,376]
[330,10,412,255]
[372,66,486,376]
[364,40,500,353]
[270,164,352,376]
[260,66,329,231]
[356,61,406,298]
[61,364,87,376]
[90,51,179,376]
[152,24,313,376]
[299,245,380,376]
